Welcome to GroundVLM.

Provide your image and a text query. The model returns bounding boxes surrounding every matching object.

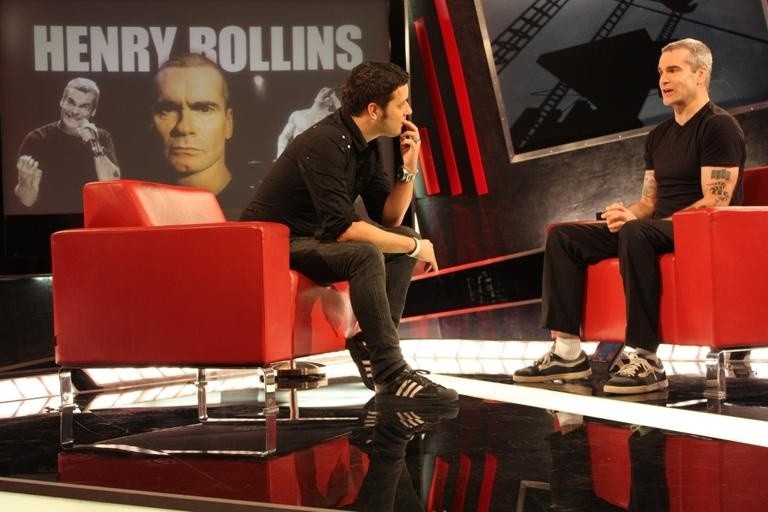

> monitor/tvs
[473,0,768,164]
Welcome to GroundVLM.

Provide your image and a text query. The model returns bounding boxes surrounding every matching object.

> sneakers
[602,351,668,394]
[347,332,374,392]
[512,349,591,383]
[374,369,459,406]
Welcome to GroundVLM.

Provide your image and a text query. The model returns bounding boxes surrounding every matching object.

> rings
[414,137,419,141]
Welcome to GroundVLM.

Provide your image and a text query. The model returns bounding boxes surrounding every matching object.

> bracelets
[404,236,421,258]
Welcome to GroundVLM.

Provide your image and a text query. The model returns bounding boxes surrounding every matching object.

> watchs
[396,165,419,185]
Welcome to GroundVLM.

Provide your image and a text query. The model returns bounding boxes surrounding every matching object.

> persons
[336,406,459,512]
[14,75,122,212]
[238,60,460,406]
[272,84,342,162]
[512,37,748,398]
[147,50,251,208]
[546,409,676,512]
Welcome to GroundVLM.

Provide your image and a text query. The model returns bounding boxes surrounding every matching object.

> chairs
[51,179,381,457]
[546,165,768,398]
[553,415,768,512]
[57,434,370,510]
